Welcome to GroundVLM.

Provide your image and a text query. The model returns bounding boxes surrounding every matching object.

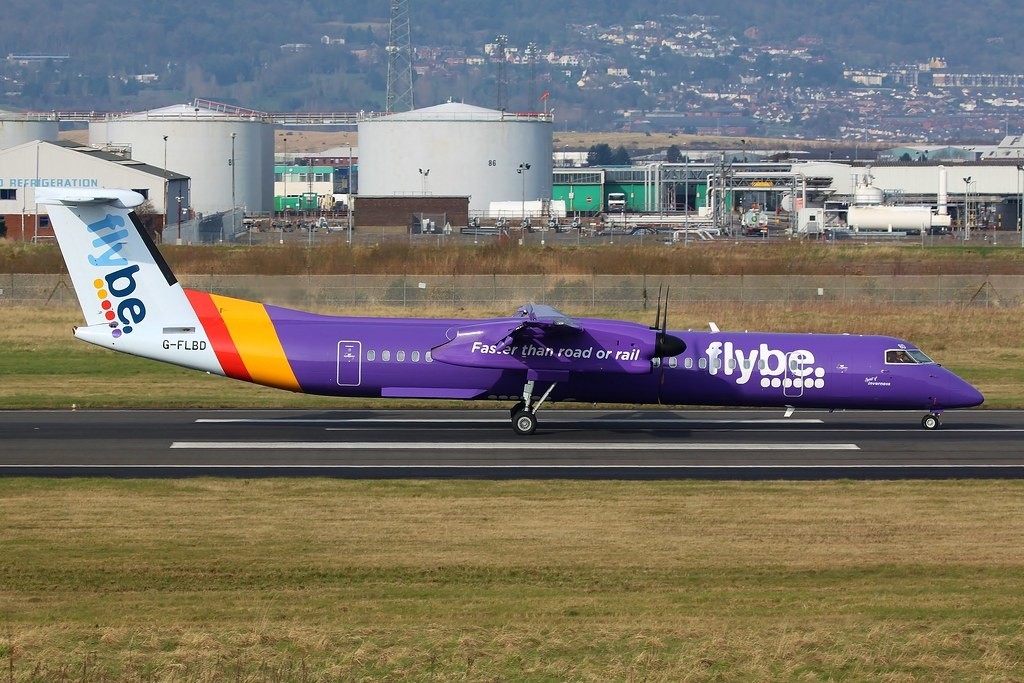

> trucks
[608,193,626,214]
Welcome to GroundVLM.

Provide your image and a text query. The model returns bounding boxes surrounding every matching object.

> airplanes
[34,185,987,437]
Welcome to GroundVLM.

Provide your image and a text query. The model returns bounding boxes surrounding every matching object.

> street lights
[517,162,531,247]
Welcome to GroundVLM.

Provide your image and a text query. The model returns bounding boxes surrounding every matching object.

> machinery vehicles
[738,207,768,238]
